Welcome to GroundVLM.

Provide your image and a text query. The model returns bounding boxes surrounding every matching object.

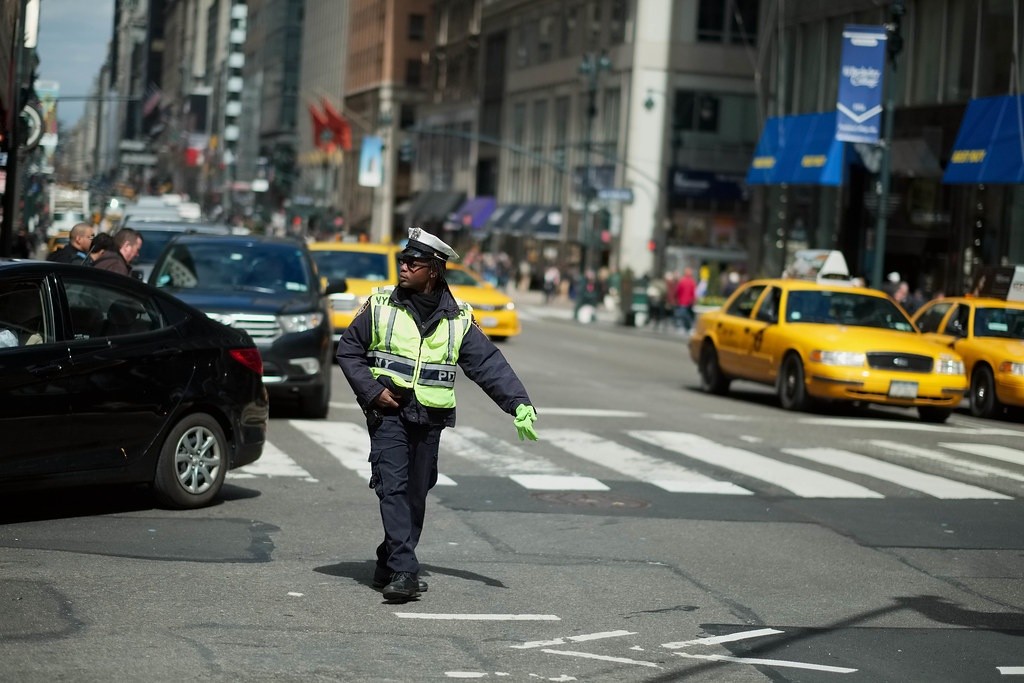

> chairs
[90,300,144,338]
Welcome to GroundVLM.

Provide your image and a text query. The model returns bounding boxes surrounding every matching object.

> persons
[335,226,537,600]
[46,222,144,281]
[720,261,1000,314]
[454,243,708,334]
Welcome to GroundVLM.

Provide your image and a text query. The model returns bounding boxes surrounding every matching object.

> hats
[400,227,460,263]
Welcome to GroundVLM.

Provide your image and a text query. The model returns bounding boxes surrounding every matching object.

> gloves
[514,403,539,442]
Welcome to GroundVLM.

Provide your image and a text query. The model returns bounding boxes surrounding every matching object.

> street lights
[645,89,683,276]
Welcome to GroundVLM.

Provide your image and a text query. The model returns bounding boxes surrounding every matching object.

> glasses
[397,257,429,269]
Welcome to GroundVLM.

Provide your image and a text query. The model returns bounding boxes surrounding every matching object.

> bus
[44,186,89,229]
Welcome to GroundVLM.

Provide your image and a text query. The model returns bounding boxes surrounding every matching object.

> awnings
[394,190,560,234]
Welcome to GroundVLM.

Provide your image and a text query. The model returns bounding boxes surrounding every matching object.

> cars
[47,231,71,255]
[910,296,1024,419]
[99,193,252,278]
[1,258,268,509]
[306,236,403,334]
[146,231,334,420]
[687,250,966,424]
[443,261,523,344]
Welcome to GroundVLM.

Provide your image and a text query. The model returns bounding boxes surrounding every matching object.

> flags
[185,132,207,168]
[38,100,59,158]
[307,96,353,154]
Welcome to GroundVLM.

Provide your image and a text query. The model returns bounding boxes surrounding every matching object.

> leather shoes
[373,565,428,592]
[382,571,422,600]
